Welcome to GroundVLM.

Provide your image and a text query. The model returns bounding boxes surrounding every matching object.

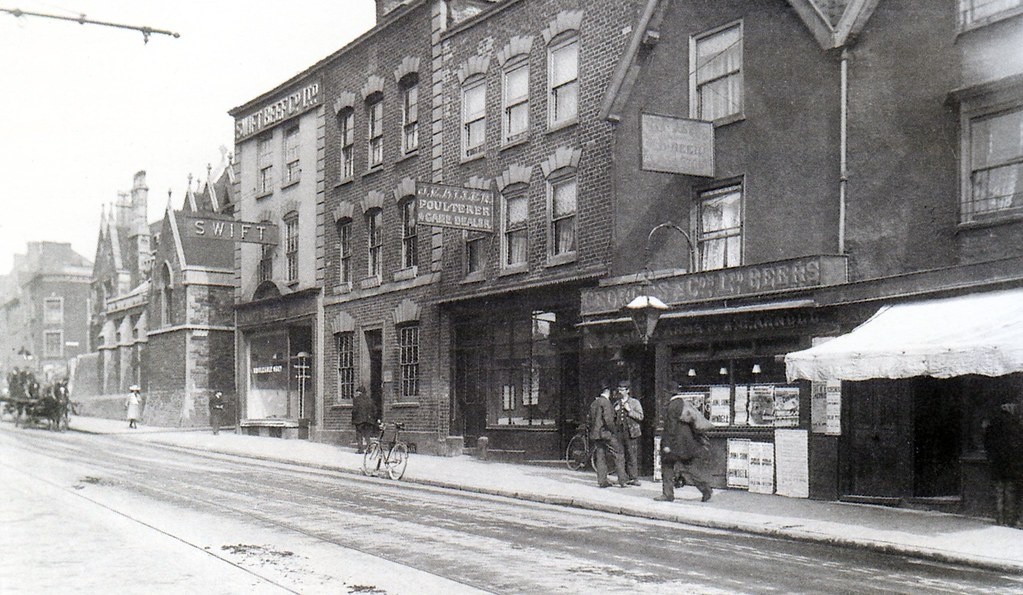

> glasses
[616,388,629,391]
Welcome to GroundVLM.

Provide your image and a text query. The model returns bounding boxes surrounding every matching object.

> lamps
[688,358,763,376]
[621,224,698,350]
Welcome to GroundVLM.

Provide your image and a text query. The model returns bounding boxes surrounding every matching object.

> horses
[2,380,71,433]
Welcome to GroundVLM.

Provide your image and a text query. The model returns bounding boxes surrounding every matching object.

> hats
[600,383,611,390]
[129,385,141,390]
[356,386,365,393]
[617,381,630,386]
[664,381,681,393]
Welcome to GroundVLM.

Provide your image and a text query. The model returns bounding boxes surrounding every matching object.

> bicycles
[362,419,411,481]
[566,423,618,475]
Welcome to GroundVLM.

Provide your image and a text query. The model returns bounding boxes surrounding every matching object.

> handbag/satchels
[680,402,715,434]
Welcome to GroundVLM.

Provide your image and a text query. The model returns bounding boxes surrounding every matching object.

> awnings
[786,288,1023,385]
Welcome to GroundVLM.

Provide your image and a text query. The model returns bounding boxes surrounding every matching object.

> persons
[351,386,378,455]
[982,399,1023,529]
[654,381,712,502]
[589,381,644,489]
[126,384,143,429]
[208,389,223,436]
[7,367,40,400]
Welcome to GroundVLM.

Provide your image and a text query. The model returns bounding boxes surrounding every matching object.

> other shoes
[701,490,714,502]
[600,483,612,488]
[631,480,641,486]
[355,451,364,454]
[653,495,675,502]
[367,450,372,454]
[621,484,631,489]
